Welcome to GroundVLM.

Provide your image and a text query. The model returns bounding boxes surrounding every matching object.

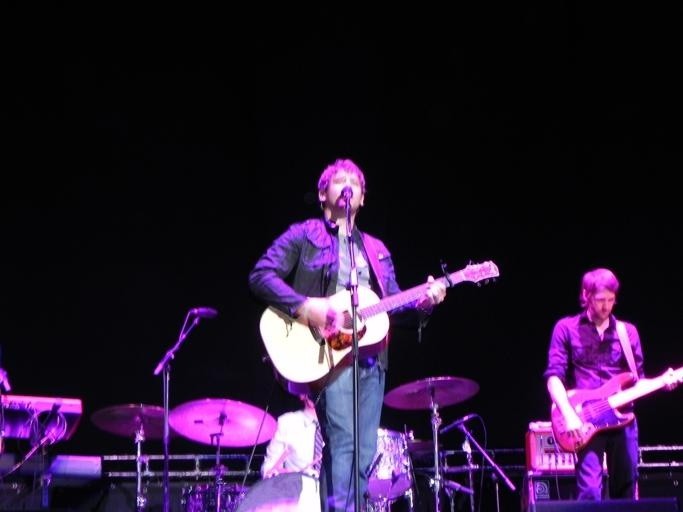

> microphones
[340,186,353,225]
[190,307,219,319]
[439,412,479,435]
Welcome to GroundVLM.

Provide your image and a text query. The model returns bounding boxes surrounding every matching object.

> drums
[364,428,415,497]
[235,472,324,512]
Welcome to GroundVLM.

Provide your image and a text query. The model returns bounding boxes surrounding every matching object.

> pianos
[0,394,85,441]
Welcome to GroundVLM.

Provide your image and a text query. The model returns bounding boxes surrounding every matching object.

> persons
[248,159,447,512]
[544,268,683,502]
[261,394,326,479]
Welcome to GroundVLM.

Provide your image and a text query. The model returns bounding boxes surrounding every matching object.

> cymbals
[91,405,169,439]
[168,399,278,447]
[383,377,478,410]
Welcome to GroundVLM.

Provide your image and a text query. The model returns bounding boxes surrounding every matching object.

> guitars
[551,366,683,452]
[259,260,501,395]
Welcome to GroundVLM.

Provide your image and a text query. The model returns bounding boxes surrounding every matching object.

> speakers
[521,475,610,511]
